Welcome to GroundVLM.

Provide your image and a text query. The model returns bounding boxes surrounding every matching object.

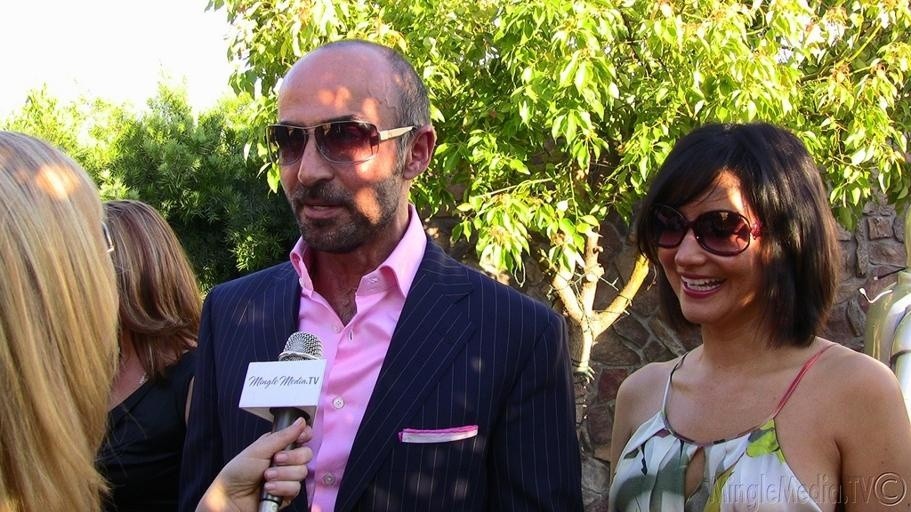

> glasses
[263,118,419,166]
[640,201,779,258]
[100,222,118,274]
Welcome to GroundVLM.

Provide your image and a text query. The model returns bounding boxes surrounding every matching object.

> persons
[173,39,584,511]
[95,199,204,512]
[1,132,316,512]
[608,120,911,512]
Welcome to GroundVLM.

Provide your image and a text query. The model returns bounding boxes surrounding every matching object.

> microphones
[239,331,324,512]
